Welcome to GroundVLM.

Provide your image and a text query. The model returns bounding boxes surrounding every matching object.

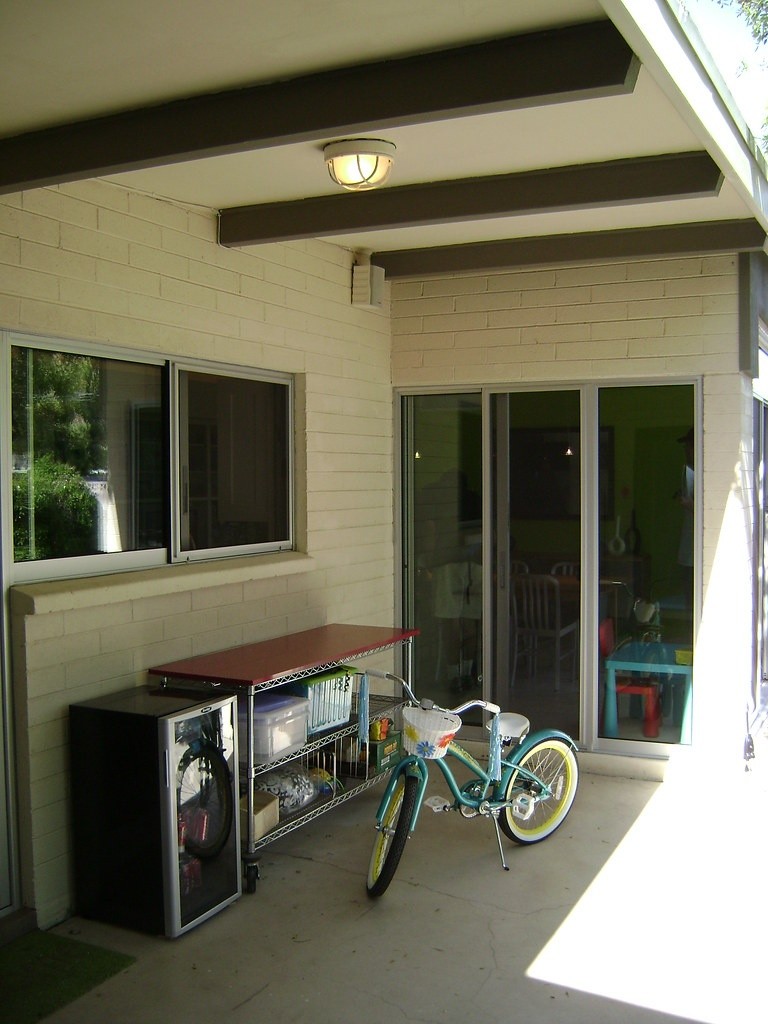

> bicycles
[365,668,580,898]
[175,707,233,859]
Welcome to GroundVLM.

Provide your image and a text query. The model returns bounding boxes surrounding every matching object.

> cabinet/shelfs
[69,683,243,939]
[161,624,422,890]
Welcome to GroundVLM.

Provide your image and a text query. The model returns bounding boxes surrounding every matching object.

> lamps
[323,140,396,192]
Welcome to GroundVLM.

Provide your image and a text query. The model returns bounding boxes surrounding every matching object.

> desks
[513,573,625,645]
[602,642,693,745]
[521,553,654,625]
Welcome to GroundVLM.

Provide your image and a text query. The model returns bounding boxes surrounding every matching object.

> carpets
[0,929,138,1024]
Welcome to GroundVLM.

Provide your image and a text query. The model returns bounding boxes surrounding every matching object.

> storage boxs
[236,694,310,764]
[331,728,402,779]
[239,790,280,842]
[288,663,357,735]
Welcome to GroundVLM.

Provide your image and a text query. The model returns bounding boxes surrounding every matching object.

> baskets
[402,706,462,759]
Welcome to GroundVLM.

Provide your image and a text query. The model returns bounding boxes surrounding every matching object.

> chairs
[508,559,580,693]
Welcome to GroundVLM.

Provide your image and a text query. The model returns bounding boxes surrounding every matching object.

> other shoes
[450,674,475,695]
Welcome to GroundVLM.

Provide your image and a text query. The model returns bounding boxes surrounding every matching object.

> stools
[603,676,663,738]
